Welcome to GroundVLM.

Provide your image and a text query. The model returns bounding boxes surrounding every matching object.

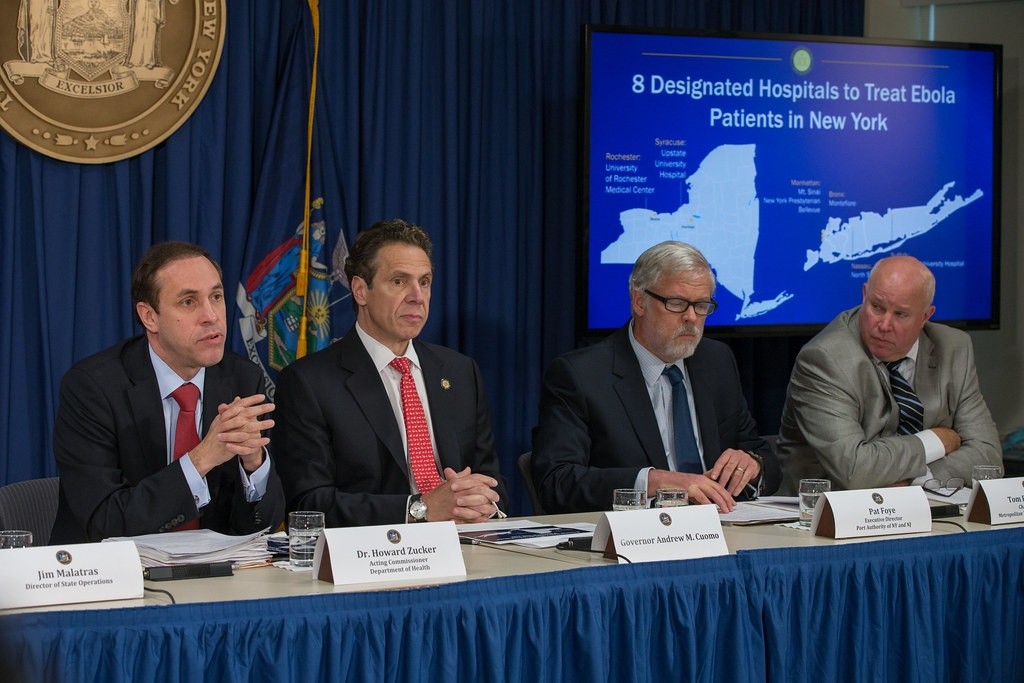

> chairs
[0,476,59,548]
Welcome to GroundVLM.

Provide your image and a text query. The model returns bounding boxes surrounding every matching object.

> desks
[0,486,1024,683]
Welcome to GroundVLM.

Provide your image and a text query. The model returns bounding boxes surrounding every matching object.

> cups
[972,465,1001,489]
[655,489,689,507]
[289,511,325,571]
[799,479,831,526]
[0,530,33,549]
[613,489,646,511]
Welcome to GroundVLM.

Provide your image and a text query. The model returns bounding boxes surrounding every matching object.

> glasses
[638,289,717,317]
[923,476,965,497]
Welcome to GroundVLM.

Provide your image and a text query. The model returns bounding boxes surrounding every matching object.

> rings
[736,467,745,472]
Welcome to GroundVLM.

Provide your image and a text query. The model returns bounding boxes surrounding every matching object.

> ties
[167,383,201,533]
[880,356,923,436]
[661,364,704,476]
[387,357,445,493]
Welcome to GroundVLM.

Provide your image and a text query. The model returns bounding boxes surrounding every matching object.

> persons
[49,241,286,545]
[763,255,1004,498]
[269,218,509,526]
[528,241,785,514]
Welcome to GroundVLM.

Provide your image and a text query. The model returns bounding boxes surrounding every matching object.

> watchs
[408,493,429,523]
